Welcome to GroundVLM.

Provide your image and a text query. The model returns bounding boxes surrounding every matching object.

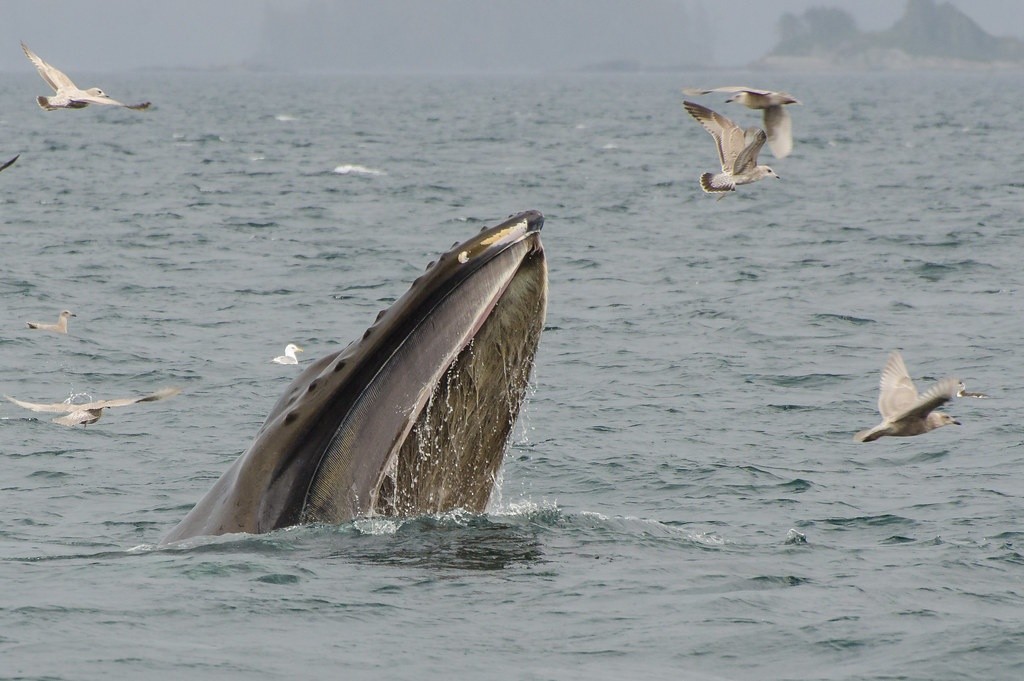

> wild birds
[684,86,801,195]
[1,153,23,171]
[22,41,151,111]
[855,351,985,443]
[26,309,77,334]
[5,388,182,428]
[272,343,303,365]
